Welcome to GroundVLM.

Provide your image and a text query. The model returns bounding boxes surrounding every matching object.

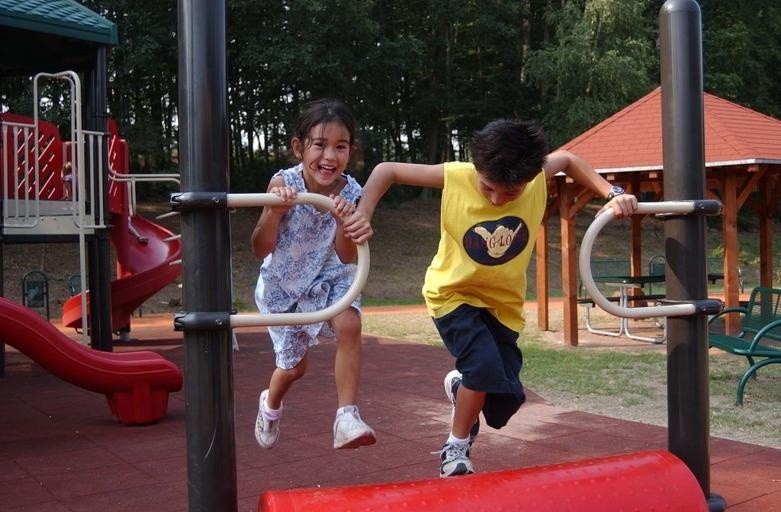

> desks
[591,273,724,343]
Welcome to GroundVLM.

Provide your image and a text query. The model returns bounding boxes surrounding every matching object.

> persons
[250,101,376,450]
[343,117,640,476]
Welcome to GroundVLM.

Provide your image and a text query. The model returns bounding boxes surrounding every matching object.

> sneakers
[254,389,284,450]
[444,369,481,443]
[439,441,476,479]
[332,405,377,450]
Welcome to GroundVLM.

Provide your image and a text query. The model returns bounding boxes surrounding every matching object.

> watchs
[607,186,625,202]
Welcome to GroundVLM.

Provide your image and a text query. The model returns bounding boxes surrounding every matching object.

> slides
[1,299,182,426]
[62,217,182,332]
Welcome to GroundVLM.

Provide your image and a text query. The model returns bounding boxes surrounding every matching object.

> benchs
[577,254,742,343]
[708,287,781,405]
